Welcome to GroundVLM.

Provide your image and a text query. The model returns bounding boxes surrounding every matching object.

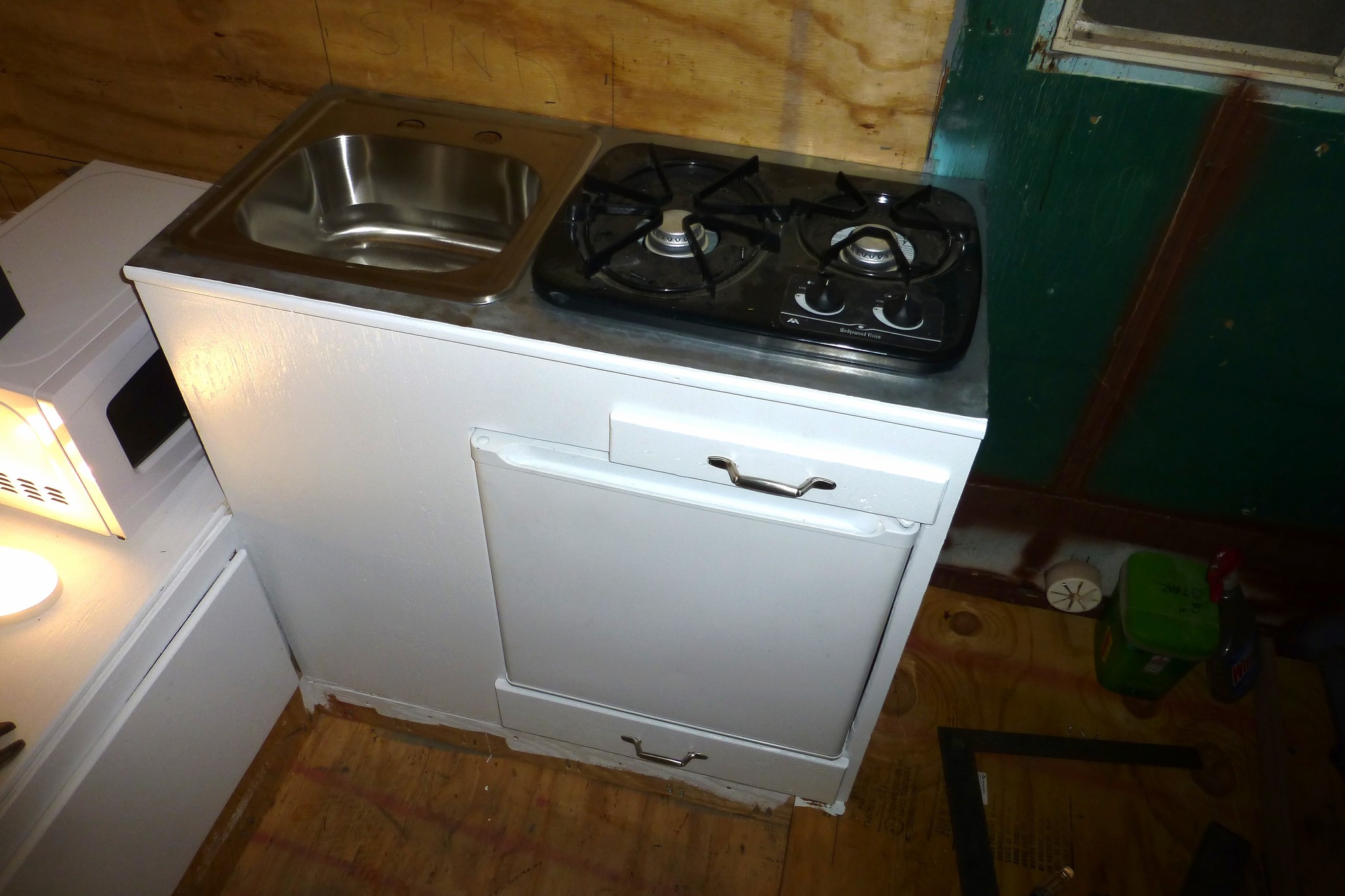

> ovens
[469,429,921,758]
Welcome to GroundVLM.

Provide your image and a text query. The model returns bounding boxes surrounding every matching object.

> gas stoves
[533,142,981,372]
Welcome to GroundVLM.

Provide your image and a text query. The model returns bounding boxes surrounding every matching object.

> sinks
[164,80,602,306]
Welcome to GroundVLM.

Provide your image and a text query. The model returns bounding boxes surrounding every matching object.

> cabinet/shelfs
[0,284,981,896]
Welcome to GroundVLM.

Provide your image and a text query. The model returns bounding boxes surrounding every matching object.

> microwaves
[0,159,225,540]
